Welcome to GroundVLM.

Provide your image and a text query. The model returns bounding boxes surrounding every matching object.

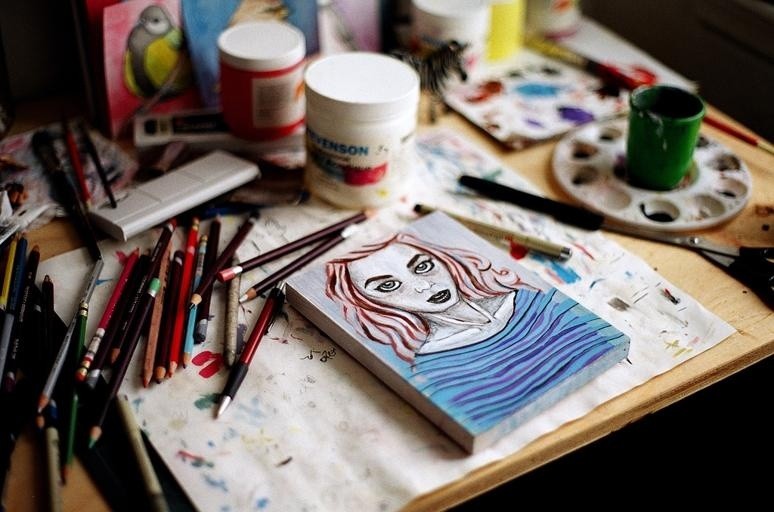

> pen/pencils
[117,393,168,512]
[413,204,571,259]
[458,175,604,231]
[214,280,285,420]
[142,214,221,387]
[31,122,119,263]
[217,207,380,304]
[78,217,176,450]
[1,232,103,510]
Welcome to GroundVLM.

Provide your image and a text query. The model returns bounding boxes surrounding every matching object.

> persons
[319,226,627,432]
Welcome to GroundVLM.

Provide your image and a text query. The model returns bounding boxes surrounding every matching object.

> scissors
[604,212,774,311]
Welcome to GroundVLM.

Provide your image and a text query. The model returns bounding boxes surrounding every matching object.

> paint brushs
[527,34,774,156]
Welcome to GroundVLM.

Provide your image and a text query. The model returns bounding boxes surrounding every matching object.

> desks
[0,90,773,512]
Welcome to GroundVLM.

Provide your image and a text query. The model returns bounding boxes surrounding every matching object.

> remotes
[131,105,233,146]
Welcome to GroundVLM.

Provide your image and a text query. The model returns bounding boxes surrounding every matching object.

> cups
[621,83,707,192]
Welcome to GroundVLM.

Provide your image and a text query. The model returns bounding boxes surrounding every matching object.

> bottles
[216,16,308,139]
[300,48,421,214]
[407,0,491,95]
